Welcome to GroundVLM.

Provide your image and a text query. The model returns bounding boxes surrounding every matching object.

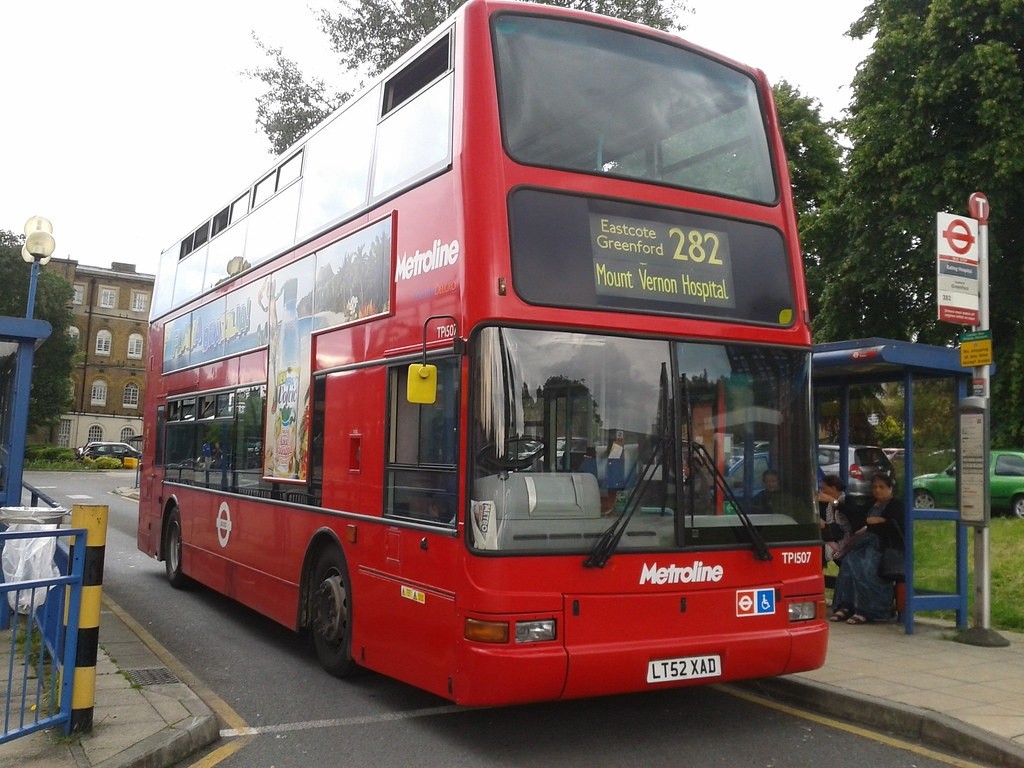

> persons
[428,417,496,521]
[683,457,906,624]
[202,441,223,469]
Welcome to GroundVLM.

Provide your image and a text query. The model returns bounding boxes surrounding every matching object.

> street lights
[22,212,57,323]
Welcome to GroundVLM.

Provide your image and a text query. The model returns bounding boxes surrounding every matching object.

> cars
[641,435,909,507]
[912,450,1024,521]
[517,434,589,471]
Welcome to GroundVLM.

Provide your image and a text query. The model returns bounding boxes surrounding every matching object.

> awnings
[811,334,996,387]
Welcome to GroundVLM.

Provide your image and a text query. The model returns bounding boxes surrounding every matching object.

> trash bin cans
[0,505,66,615]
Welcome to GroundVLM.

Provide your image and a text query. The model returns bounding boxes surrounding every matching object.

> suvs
[80,441,142,471]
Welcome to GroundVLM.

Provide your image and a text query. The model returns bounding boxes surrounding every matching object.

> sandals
[846,612,871,625]
[830,608,852,622]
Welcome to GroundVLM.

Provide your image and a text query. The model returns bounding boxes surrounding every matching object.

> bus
[135,1,829,707]
